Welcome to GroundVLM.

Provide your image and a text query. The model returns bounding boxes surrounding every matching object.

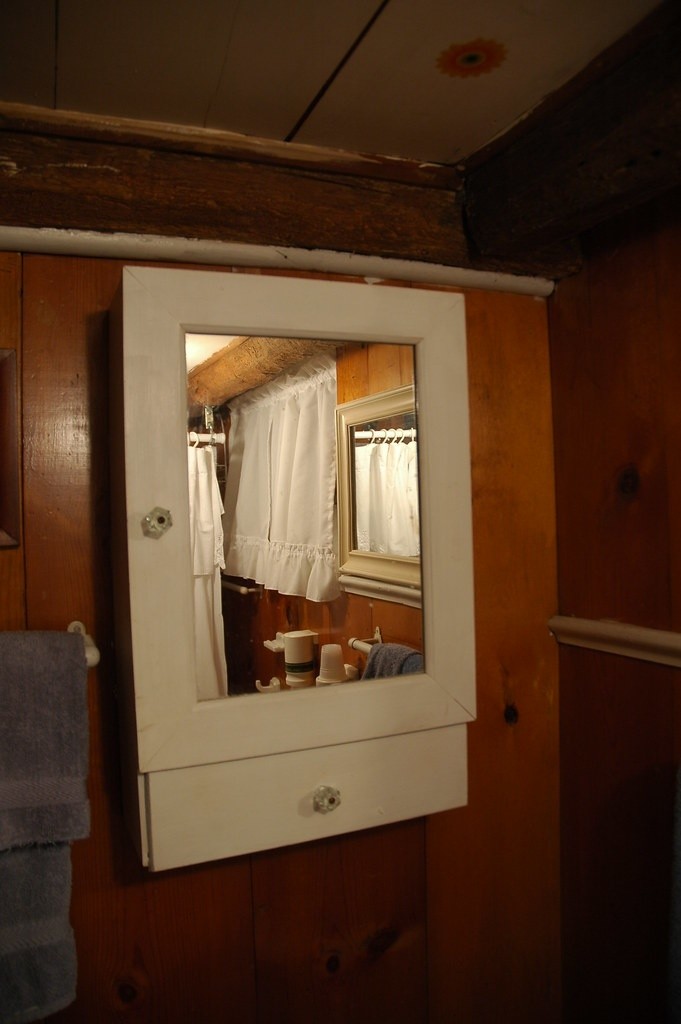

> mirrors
[335,383,422,610]
[122,266,478,773]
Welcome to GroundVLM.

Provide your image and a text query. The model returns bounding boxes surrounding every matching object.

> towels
[361,641,423,682]
[0,626,92,1024]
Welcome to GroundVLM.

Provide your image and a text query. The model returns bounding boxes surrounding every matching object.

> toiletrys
[283,628,351,689]
